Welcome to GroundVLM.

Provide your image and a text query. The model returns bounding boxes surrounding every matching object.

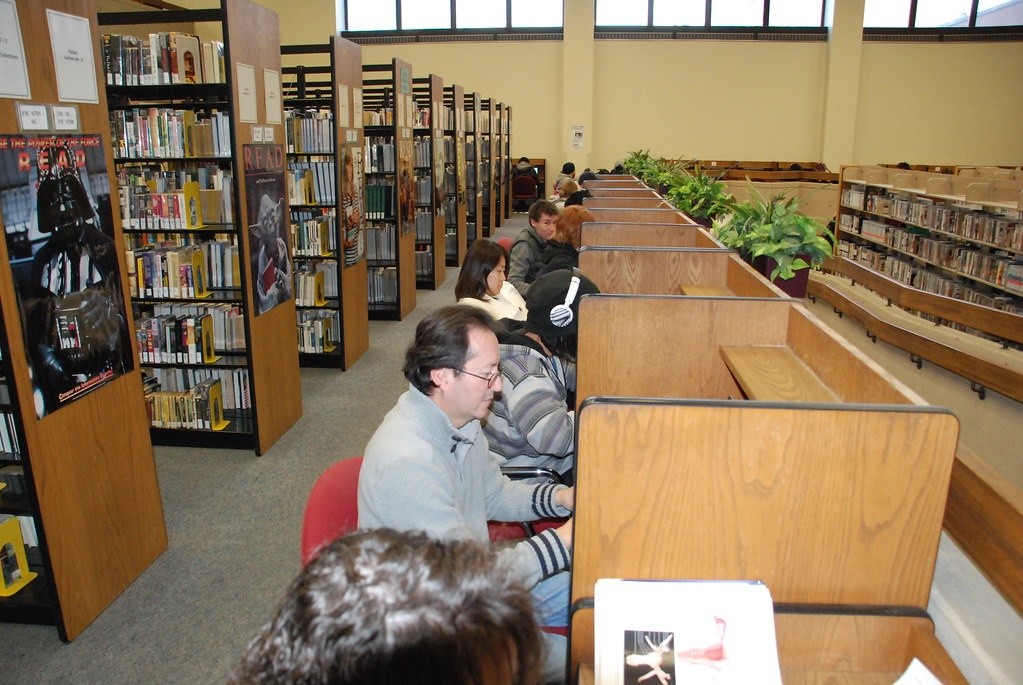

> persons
[575,133,580,138]
[341,147,361,267]
[896,162,918,171]
[24,137,123,418]
[821,215,836,253]
[506,199,595,297]
[250,194,291,312]
[356,304,575,685]
[789,163,803,171]
[230,528,544,685]
[554,160,608,208]
[455,239,601,485]
[513,157,537,202]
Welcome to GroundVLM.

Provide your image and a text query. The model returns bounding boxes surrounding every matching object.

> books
[282,105,341,354]
[102,31,253,430]
[0,351,48,599]
[832,189,1023,351]
[483,115,508,206]
[363,106,474,304]
[593,578,781,685]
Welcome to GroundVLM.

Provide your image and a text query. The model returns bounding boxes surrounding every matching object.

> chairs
[513,176,538,201]
[302,457,570,637]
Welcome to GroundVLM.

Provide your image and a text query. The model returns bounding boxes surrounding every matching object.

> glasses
[441,366,504,389]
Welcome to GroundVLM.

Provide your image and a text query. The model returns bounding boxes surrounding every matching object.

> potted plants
[619,147,736,228]
[710,176,837,298]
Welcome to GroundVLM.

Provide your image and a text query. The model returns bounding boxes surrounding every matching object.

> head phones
[547,266,582,328]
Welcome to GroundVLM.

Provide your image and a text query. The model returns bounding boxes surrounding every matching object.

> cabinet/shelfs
[0,0,513,647]
[829,165,1023,353]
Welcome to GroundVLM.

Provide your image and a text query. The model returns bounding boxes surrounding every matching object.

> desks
[569,173,973,685]
[512,158,546,194]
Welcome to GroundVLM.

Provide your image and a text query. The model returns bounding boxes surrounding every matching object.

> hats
[524,268,600,337]
